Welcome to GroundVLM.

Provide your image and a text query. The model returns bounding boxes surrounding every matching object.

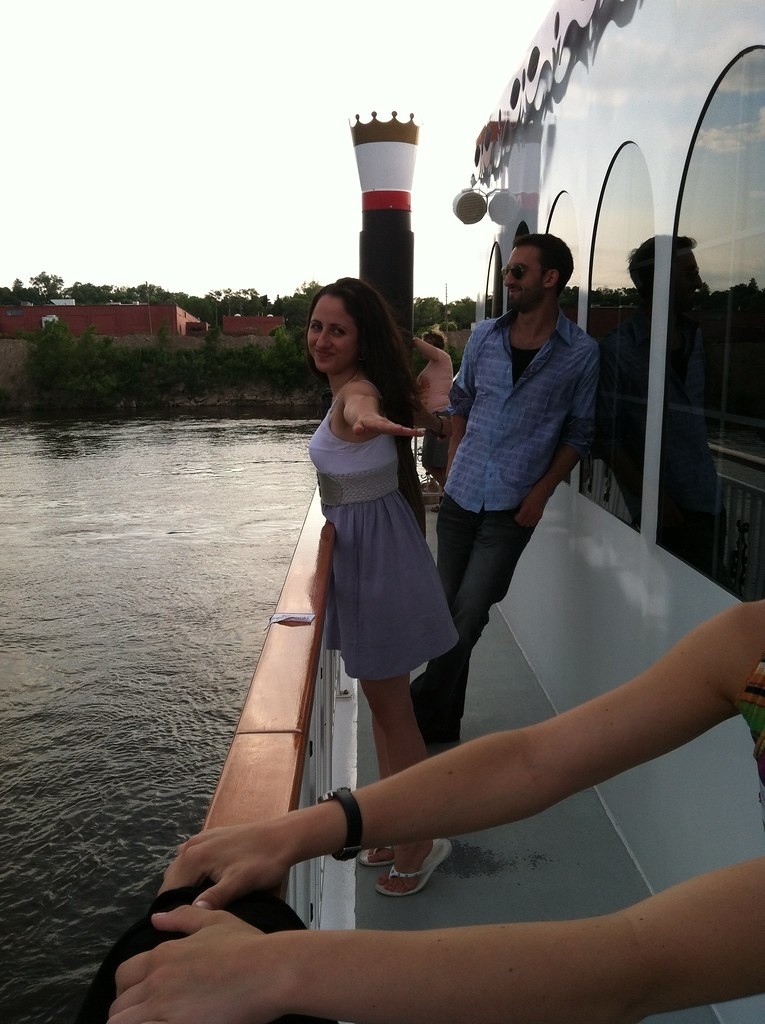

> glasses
[501,263,553,280]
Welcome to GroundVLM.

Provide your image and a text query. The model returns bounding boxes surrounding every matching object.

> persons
[106,598,765,1024]
[601,237,726,583]
[308,278,460,895]
[412,331,456,512]
[409,237,602,744]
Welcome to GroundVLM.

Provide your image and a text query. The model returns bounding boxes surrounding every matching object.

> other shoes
[421,730,462,744]
[431,505,440,512]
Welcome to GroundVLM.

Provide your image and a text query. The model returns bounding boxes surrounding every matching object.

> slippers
[375,837,453,895]
[357,845,395,866]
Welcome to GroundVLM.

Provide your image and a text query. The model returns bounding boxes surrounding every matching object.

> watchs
[317,787,364,861]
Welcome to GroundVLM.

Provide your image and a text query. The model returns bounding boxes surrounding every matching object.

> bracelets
[437,416,444,434]
